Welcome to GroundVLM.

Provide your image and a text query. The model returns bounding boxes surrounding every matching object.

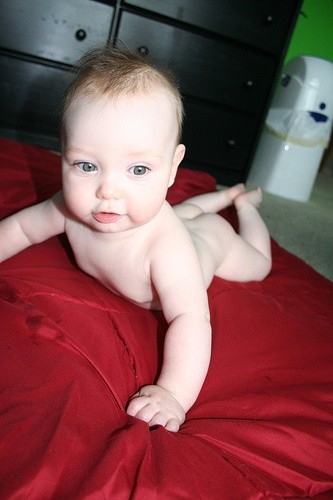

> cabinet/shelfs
[0,0,300,191]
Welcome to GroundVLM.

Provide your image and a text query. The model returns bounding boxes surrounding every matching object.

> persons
[0,46,272,433]
